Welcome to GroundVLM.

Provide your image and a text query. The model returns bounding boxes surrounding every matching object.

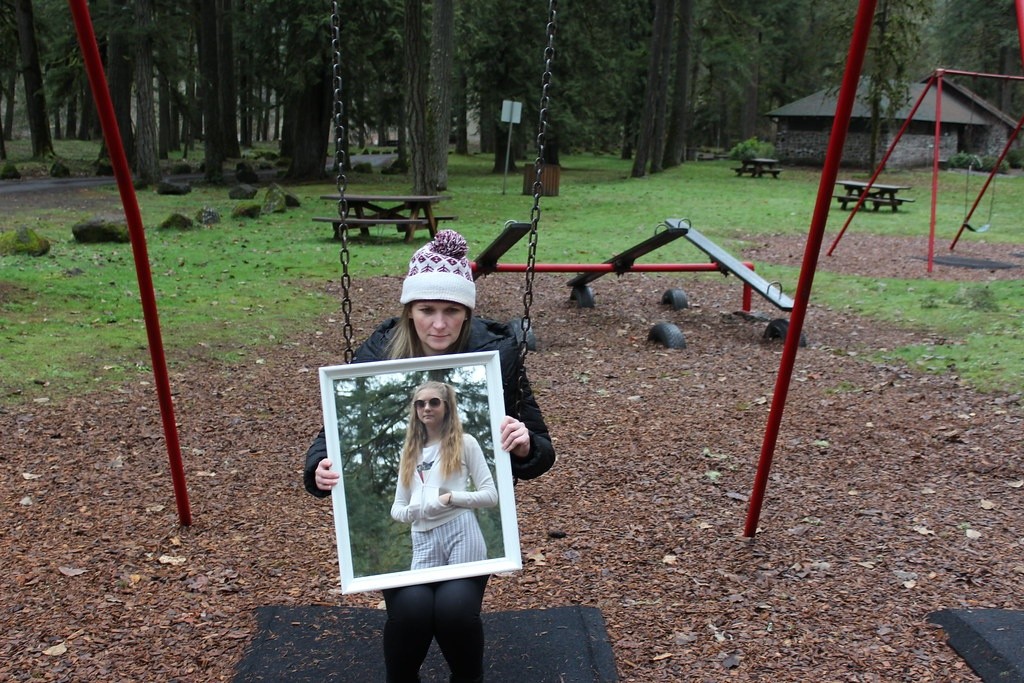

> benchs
[312,213,459,232]
[832,194,915,208]
[730,167,783,175]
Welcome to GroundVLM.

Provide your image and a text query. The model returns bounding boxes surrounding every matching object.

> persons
[303,229,556,683]
[390,381,498,571]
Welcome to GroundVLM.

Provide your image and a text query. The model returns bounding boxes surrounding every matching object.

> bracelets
[448,494,452,504]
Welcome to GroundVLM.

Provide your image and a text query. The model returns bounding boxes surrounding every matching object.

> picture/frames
[318,350,523,595]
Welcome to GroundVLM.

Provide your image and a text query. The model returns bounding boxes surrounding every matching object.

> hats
[399,229,476,311]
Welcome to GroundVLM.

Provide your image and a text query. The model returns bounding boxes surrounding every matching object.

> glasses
[413,398,448,410]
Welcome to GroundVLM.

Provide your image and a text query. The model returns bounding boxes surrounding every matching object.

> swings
[963,78,1002,233]
[318,1,557,492]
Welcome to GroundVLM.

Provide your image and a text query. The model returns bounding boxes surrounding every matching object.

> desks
[835,181,912,212]
[320,195,444,243]
[738,160,779,178]
